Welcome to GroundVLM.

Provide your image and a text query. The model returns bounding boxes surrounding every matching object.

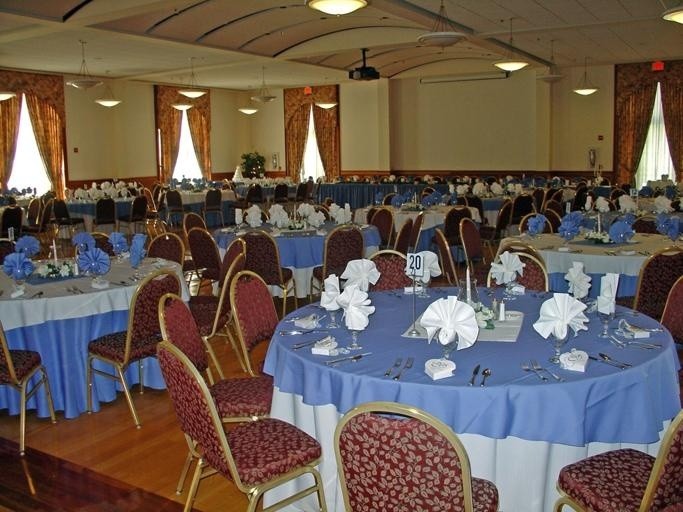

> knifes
[639,251,647,256]
[121,281,132,286]
[279,331,315,335]
[588,356,626,370]
[468,364,481,386]
[326,353,372,364]
[292,340,317,349]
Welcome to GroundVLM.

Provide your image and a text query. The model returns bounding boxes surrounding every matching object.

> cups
[347,316,365,350]
[418,270,431,298]
[547,324,569,364]
[437,329,459,360]
[597,301,616,338]
[502,271,517,300]
[324,293,340,329]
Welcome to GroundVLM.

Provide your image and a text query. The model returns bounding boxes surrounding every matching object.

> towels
[328,203,340,218]
[108,232,128,254]
[3,253,35,279]
[374,192,384,201]
[527,217,539,236]
[532,293,589,339]
[341,259,381,292]
[335,208,352,225]
[595,197,610,212]
[490,251,526,285]
[431,191,442,203]
[655,212,680,241]
[451,192,457,205]
[245,204,263,228]
[320,274,341,311]
[618,194,638,213]
[420,296,479,350]
[297,203,315,216]
[78,249,111,275]
[563,211,583,226]
[268,204,288,228]
[15,236,40,256]
[585,196,592,211]
[307,211,326,227]
[608,221,634,243]
[236,209,243,224]
[404,251,442,284]
[403,192,413,202]
[558,222,579,241]
[536,213,546,232]
[421,196,433,208]
[391,195,405,208]
[654,196,675,214]
[72,232,96,250]
[130,234,148,268]
[336,285,376,330]
[597,273,619,315]
[565,261,592,298]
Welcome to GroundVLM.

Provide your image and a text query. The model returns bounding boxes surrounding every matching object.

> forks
[385,358,403,376]
[520,360,548,382]
[392,356,414,381]
[610,334,663,349]
[72,285,84,294]
[529,359,561,380]
[608,338,654,350]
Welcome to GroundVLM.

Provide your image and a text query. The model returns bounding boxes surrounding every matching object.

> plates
[621,250,636,256]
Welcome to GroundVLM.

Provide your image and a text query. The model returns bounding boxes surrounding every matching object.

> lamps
[535,40,565,84]
[178,55,208,98]
[347,49,379,81]
[316,76,338,110]
[94,68,122,108]
[418,73,508,83]
[572,57,599,96]
[309,0,368,16]
[66,40,98,92]
[661,4,683,25]
[171,102,194,110]
[251,66,277,103]
[238,84,259,115]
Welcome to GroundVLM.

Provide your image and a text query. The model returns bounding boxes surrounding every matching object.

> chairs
[166,190,196,229]
[416,2,468,50]
[630,215,663,235]
[188,227,222,296]
[432,207,472,275]
[591,177,610,187]
[265,183,289,209]
[91,198,116,232]
[116,196,148,245]
[288,183,307,206]
[243,229,298,316]
[411,211,425,254]
[335,402,499,512]
[434,228,460,287]
[158,293,273,492]
[187,254,246,380]
[0,322,57,502]
[661,276,683,344]
[219,237,247,299]
[422,187,434,204]
[22,201,52,235]
[492,17,530,74]
[371,209,395,249]
[393,219,413,254]
[635,246,683,319]
[53,200,86,239]
[486,177,496,185]
[520,213,554,235]
[512,193,537,232]
[201,189,225,228]
[551,189,564,203]
[552,415,681,509]
[139,187,166,235]
[621,183,631,195]
[229,183,266,209]
[309,225,364,303]
[368,250,414,292]
[0,207,22,262]
[230,271,281,378]
[544,209,563,233]
[572,186,589,212]
[87,268,182,429]
[43,193,54,206]
[456,197,470,207]
[593,198,616,213]
[459,218,498,275]
[27,199,40,227]
[610,188,627,210]
[533,188,545,212]
[157,340,327,512]
[488,252,549,292]
[480,200,511,261]
[146,232,186,267]
[382,193,397,205]
[547,199,564,220]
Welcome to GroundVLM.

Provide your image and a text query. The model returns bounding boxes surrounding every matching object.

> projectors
[349,67,378,81]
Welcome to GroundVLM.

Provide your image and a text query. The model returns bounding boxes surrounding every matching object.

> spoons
[66,286,78,294]
[480,368,491,386]
[599,353,632,367]
